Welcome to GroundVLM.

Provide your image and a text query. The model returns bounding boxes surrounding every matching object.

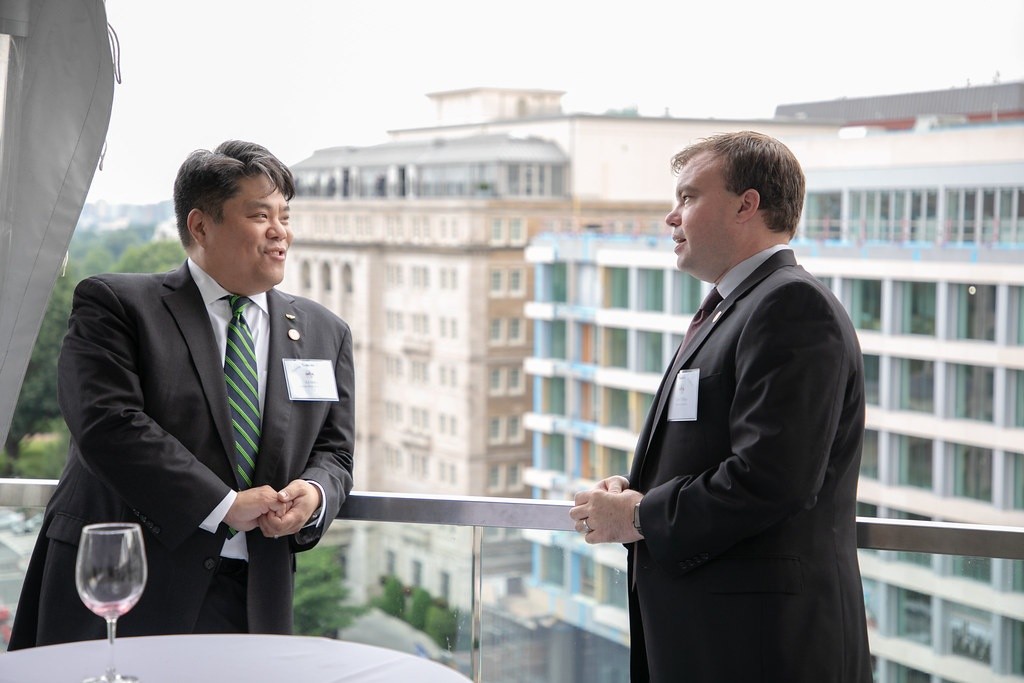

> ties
[219,294,261,541]
[671,288,724,367]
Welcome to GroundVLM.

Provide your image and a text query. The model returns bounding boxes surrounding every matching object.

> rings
[582,518,592,532]
[274,535,278,538]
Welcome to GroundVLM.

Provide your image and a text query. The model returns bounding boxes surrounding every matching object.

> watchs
[309,506,322,523]
[633,503,644,536]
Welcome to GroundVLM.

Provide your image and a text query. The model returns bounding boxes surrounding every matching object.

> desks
[2,635,473,683]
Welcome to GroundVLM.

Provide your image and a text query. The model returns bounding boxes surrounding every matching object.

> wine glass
[74,522,148,683]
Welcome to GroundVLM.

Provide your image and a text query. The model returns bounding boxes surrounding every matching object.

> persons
[570,131,873,683]
[6,140,355,654]
